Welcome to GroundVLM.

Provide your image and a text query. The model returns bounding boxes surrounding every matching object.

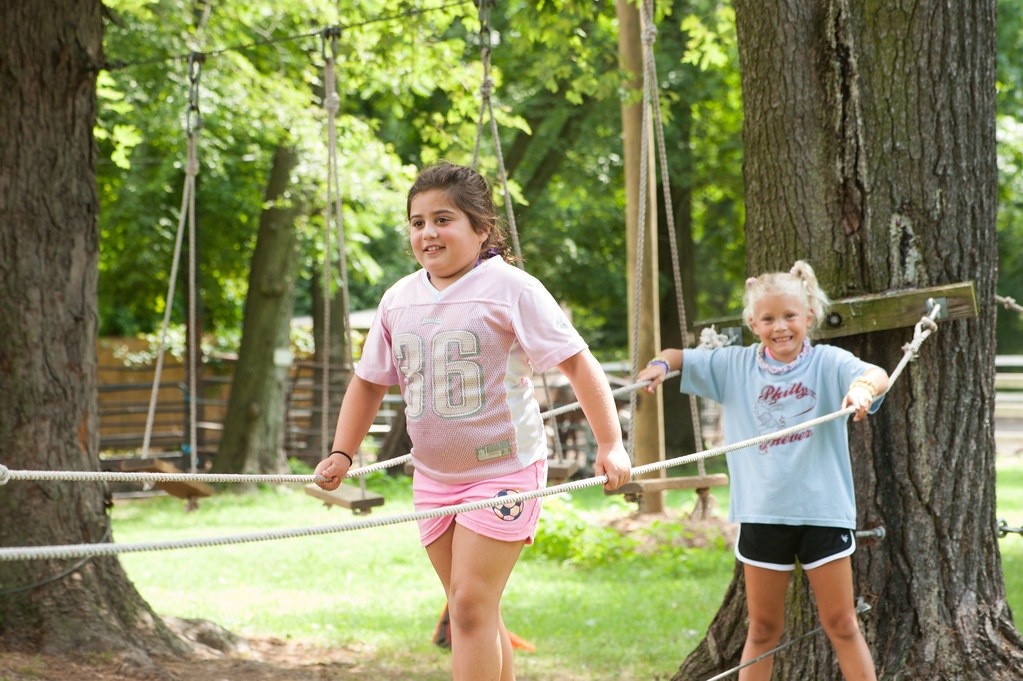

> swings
[602,1,729,494]
[305,56,383,509]
[122,134,213,499]
[403,49,577,480]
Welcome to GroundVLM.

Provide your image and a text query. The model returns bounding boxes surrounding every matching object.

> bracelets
[327,450,354,468]
[647,356,671,376]
[848,376,879,398]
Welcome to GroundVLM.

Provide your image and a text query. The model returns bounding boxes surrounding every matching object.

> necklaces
[757,335,812,376]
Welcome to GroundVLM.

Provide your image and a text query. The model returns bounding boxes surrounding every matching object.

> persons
[634,258,893,681]
[311,158,637,681]
